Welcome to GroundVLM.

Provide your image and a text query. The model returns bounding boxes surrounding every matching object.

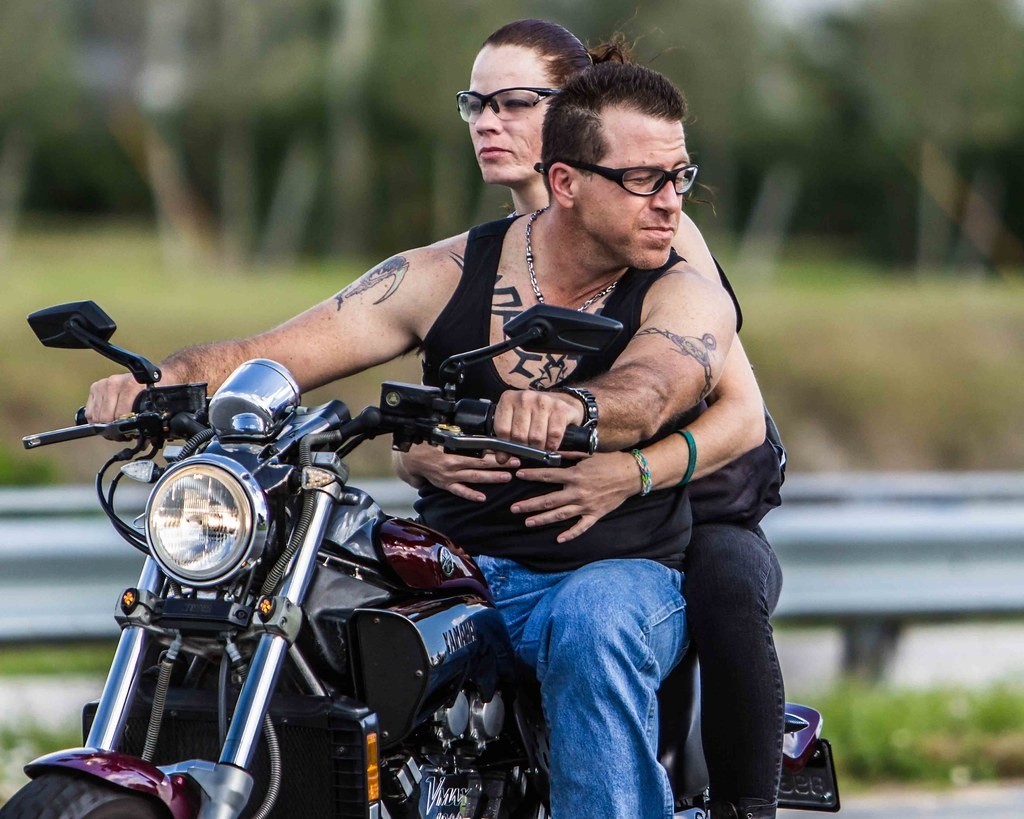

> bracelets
[676,429,697,485]
[631,449,652,496]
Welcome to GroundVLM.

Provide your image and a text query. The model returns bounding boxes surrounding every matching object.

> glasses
[455,87,561,122]
[533,161,700,197]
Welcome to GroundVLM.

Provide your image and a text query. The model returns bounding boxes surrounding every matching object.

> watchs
[551,386,599,430]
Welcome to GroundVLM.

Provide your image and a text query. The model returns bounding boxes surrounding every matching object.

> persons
[84,60,736,819]
[391,19,786,819]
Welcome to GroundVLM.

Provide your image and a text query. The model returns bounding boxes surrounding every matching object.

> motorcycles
[0,301,844,819]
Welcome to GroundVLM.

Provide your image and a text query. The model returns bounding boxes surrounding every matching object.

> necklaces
[525,208,618,313]
